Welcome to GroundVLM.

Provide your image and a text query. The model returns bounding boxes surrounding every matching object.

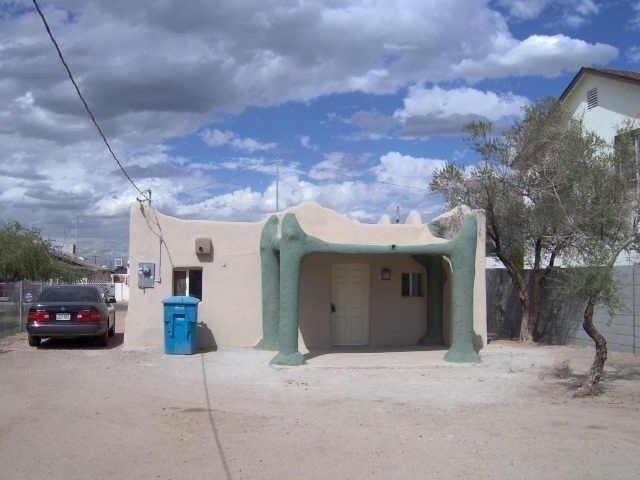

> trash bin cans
[161,296,200,355]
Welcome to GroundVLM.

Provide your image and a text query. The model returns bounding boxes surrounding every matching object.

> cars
[26,283,116,346]
